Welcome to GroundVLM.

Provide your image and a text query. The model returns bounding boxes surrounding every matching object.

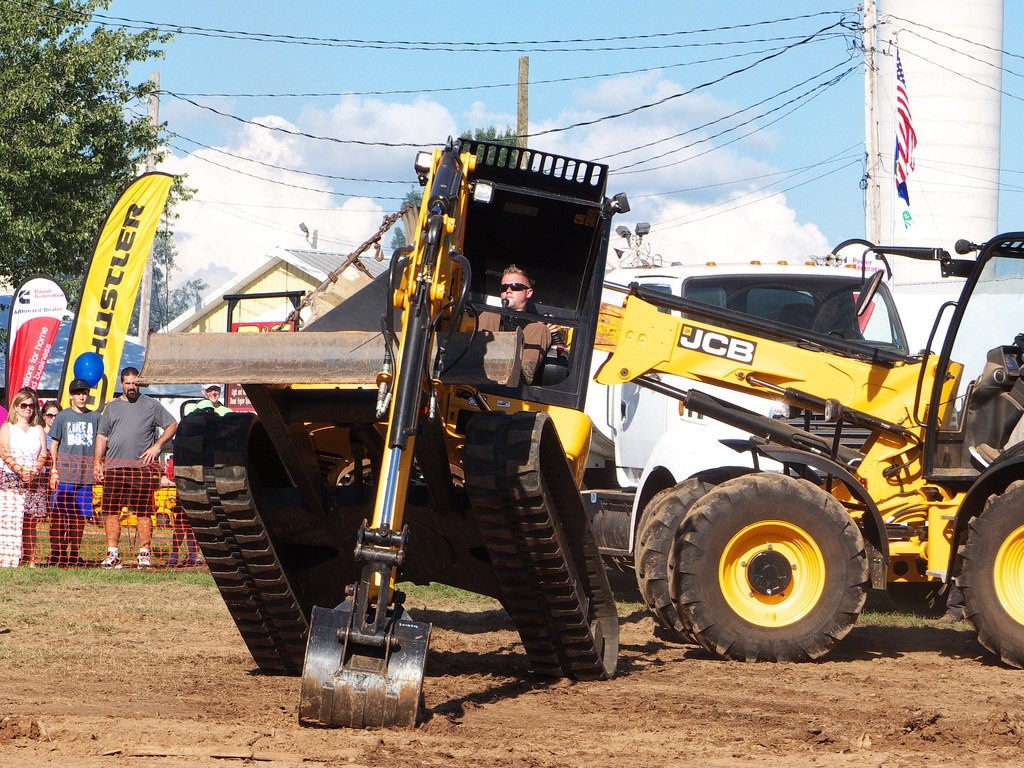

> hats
[69,379,90,393]
[202,383,221,390]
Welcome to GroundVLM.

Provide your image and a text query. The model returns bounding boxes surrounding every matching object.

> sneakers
[101,551,123,570]
[137,551,152,570]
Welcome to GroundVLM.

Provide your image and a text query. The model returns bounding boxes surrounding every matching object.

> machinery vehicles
[591,227,1023,668]
[127,134,632,734]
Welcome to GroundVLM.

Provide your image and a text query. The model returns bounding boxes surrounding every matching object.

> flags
[889,49,918,208]
[56,169,176,414]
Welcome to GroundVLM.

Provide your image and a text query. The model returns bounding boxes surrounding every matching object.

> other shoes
[520,362,535,385]
[976,443,1005,463]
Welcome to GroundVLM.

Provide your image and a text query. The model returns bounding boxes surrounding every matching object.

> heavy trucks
[573,256,910,491]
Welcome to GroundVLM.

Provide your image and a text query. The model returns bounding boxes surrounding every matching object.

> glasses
[18,403,36,409]
[42,413,57,419]
[206,388,220,392]
[498,283,530,293]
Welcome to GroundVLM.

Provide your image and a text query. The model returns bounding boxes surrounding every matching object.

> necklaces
[41,378,103,568]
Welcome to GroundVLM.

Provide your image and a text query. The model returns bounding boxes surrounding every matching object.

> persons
[477,261,564,386]
[163,399,215,567]
[199,382,234,416]
[93,366,178,573]
[38,400,64,454]
[0,385,49,566]
